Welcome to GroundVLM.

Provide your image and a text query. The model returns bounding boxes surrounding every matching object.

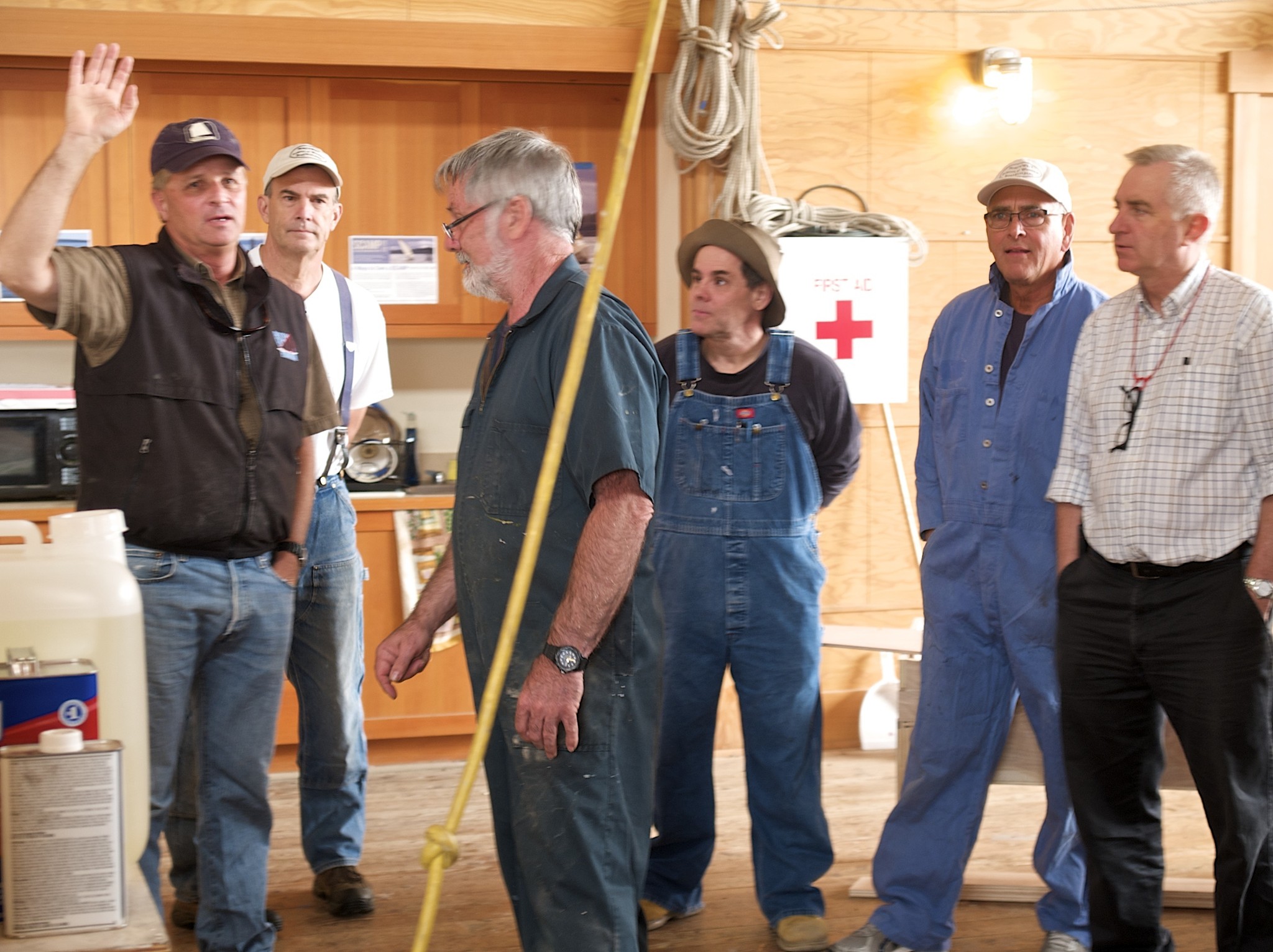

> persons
[373,128,678,952]
[1048,143,1273,951]
[828,156,1111,951]
[0,39,346,952]
[627,219,866,952]
[161,139,397,929]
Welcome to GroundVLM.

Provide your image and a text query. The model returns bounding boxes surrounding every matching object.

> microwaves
[1,387,86,500]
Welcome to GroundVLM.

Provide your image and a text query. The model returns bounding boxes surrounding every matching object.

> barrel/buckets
[1,503,152,940]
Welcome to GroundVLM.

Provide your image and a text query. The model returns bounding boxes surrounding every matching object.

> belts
[1091,540,1255,579]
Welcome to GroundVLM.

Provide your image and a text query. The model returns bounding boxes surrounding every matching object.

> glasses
[1108,386,1142,453]
[442,196,506,241]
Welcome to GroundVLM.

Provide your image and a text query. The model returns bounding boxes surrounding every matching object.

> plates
[346,437,398,483]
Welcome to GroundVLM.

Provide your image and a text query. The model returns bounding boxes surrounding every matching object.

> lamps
[975,45,1033,124]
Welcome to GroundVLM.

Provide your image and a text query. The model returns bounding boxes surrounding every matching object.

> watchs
[1241,576,1273,603]
[541,640,589,677]
[274,539,309,569]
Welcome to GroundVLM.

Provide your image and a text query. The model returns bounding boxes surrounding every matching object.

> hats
[679,218,785,329]
[978,157,1073,214]
[263,143,343,200]
[151,117,250,176]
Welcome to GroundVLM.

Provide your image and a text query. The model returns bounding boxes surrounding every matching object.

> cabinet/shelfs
[276,511,477,726]
[0,0,685,345]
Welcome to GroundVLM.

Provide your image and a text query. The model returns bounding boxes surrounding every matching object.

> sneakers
[776,916,830,951]
[1041,929,1090,952]
[171,898,283,932]
[637,898,674,931]
[826,922,918,952]
[311,866,375,916]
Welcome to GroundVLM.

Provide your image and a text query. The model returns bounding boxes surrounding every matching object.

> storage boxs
[771,236,908,406]
